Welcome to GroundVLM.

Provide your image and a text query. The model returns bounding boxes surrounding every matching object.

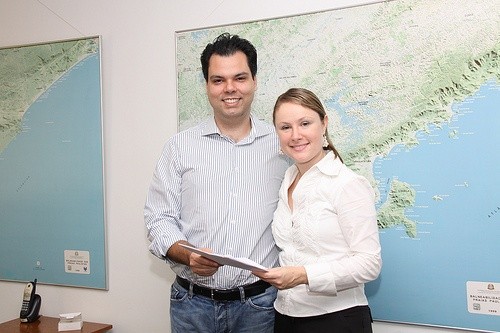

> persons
[252,88,382,333]
[143,33,295,333]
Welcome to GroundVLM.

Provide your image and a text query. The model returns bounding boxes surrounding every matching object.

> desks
[0,316,112,333]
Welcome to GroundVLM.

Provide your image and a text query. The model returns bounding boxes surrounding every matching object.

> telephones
[20,279,41,322]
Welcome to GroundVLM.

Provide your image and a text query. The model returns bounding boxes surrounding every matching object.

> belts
[176,275,272,301]
